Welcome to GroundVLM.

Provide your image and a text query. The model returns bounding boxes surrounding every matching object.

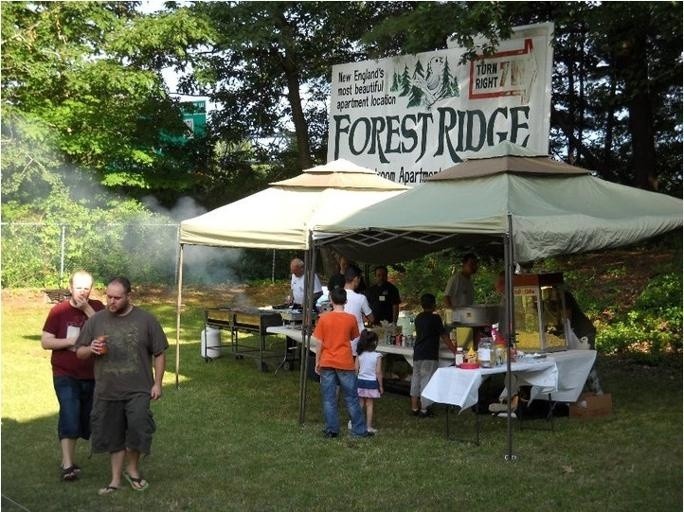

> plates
[460,364,479,369]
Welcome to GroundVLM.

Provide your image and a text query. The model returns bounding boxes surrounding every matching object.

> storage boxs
[570,393,613,416]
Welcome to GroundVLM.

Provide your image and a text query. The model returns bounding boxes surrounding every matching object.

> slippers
[121,469,149,492]
[96,486,118,497]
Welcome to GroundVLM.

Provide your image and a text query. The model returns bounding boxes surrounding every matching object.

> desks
[376,342,512,384]
[439,350,596,446]
[266,321,359,383]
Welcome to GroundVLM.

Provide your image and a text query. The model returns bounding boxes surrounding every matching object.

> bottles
[478,337,507,368]
[387,329,417,348]
[97,336,108,355]
[455,346,464,368]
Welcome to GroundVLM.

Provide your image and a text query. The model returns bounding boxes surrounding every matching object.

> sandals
[61,461,82,479]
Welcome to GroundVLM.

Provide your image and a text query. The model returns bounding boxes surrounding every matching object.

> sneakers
[324,409,433,438]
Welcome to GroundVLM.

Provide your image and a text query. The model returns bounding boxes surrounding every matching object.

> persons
[284,257,322,362]
[365,266,401,325]
[328,256,366,295]
[75,277,169,497]
[342,263,375,335]
[311,284,376,438]
[443,252,477,309]
[348,328,384,433]
[534,285,602,393]
[40,270,108,481]
[408,293,456,416]
[497,261,534,293]
